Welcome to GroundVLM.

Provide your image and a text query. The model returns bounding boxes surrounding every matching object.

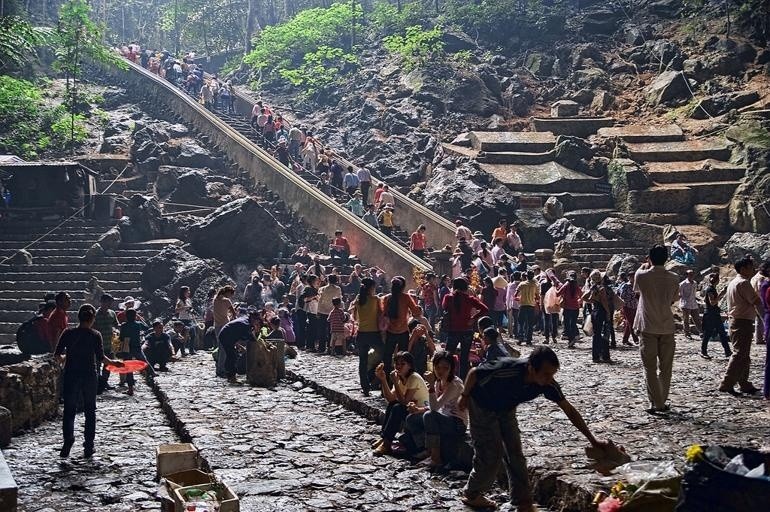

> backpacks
[17,316,42,354]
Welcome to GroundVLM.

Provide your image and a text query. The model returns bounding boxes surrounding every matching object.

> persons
[405,351,476,468]
[55,302,126,459]
[456,345,607,512]
[699,272,729,358]
[677,269,703,340]
[748,262,770,344]
[759,256,769,398]
[370,352,431,456]
[212,246,645,397]
[109,39,237,115]
[718,258,767,395]
[409,221,521,261]
[670,234,700,266]
[250,100,395,236]
[632,244,682,412]
[33,276,217,398]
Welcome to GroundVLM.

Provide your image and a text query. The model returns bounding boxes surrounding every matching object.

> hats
[491,275,508,289]
[118,296,142,309]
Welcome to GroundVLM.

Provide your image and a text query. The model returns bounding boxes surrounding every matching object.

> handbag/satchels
[439,310,451,333]
[377,311,390,331]
[612,294,625,312]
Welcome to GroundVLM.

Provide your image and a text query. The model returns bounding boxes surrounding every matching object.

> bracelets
[460,390,469,397]
[429,387,435,394]
